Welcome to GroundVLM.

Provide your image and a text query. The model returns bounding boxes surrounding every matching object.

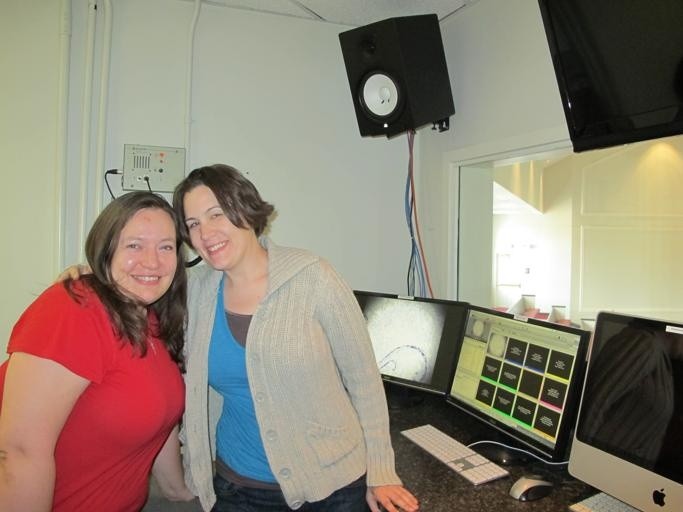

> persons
[57,164,419,512]
[0,190,200,512]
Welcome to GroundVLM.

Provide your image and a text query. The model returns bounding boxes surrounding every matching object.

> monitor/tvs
[351,290,469,410]
[444,305,592,467]
[567,310,683,512]
[538,0,683,153]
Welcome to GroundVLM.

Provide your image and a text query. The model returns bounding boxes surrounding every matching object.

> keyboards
[399,423,510,486]
[569,492,643,512]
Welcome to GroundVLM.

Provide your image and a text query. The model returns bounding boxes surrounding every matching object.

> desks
[375,388,594,511]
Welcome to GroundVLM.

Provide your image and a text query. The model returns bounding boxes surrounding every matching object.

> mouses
[509,473,555,502]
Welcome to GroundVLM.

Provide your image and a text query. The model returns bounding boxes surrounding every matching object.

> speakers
[339,15,455,138]
